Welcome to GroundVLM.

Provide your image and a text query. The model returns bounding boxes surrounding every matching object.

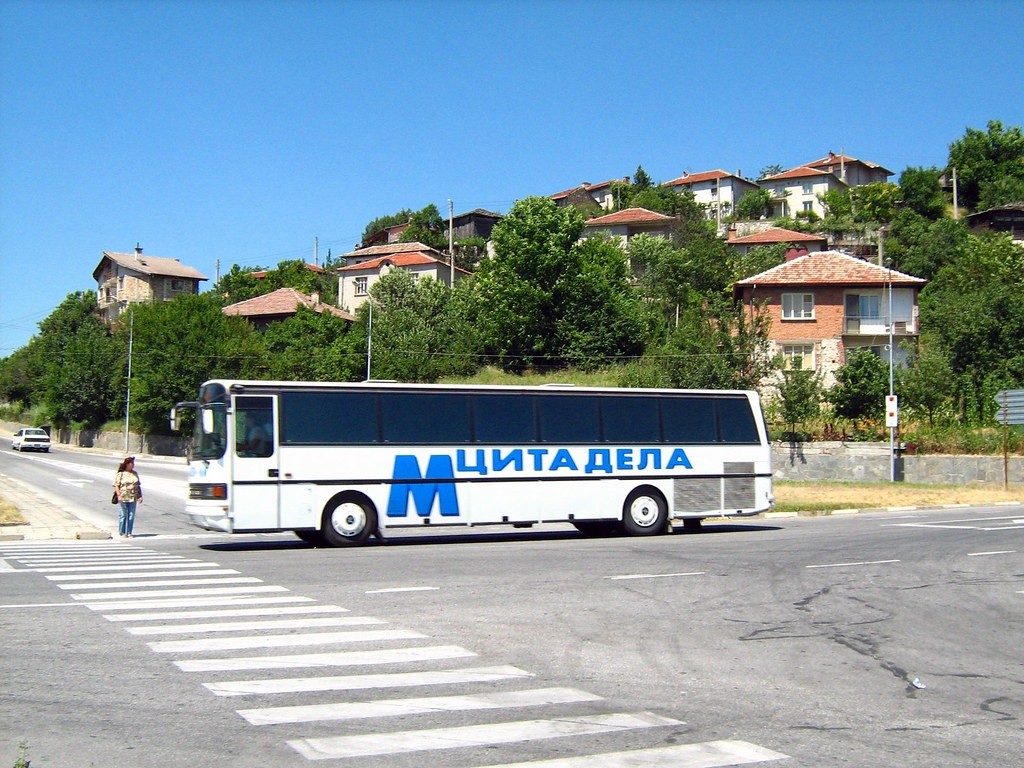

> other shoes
[120,534,133,539]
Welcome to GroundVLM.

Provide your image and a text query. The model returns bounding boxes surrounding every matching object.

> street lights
[883,255,896,484]
[350,280,372,383]
[109,293,134,454]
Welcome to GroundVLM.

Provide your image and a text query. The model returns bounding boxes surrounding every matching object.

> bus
[170,379,776,549]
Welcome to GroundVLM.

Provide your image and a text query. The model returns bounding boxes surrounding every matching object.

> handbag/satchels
[112,491,118,504]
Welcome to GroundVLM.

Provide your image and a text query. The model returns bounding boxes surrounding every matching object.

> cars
[12,428,51,453]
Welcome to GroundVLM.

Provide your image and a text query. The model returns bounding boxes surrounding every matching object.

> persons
[237,414,264,452]
[114,457,143,538]
